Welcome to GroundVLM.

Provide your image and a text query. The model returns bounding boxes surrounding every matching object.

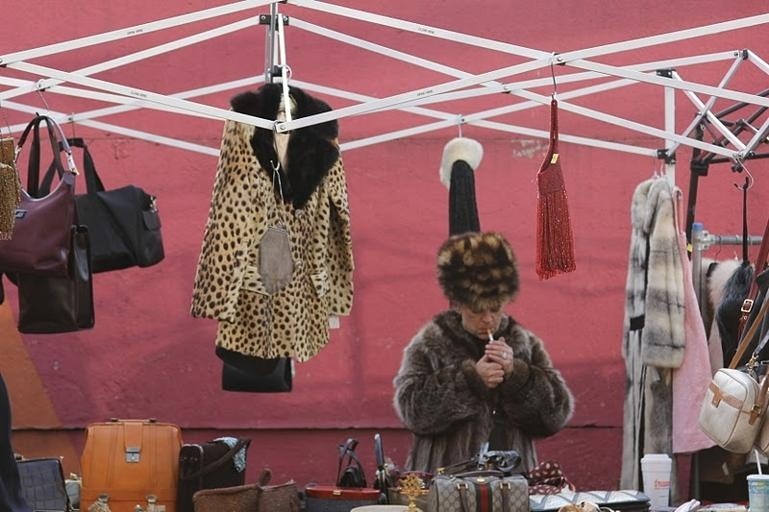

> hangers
[651,151,683,201]
[709,234,752,264]
[264,65,300,112]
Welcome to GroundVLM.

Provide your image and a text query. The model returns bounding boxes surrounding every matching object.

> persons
[391,230,576,474]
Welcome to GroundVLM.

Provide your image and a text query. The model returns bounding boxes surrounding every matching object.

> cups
[640,454,672,511]
[746,474,769,512]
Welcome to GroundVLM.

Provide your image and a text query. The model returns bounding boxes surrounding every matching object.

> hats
[437,231,519,311]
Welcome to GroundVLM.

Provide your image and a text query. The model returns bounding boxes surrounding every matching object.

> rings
[501,351,506,358]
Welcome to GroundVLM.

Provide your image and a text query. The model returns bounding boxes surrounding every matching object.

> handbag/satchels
[12,454,73,512]
[304,438,380,512]
[529,489,651,512]
[387,471,436,512]
[175,437,251,512]
[373,433,401,505]
[696,221,769,465]
[427,442,529,512]
[192,468,299,512]
[258,171,293,281]
[1,115,165,336]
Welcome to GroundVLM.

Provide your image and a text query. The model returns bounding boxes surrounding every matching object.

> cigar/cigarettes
[487,328,494,341]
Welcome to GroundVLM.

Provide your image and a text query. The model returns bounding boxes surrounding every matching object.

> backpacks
[80,416,184,512]
